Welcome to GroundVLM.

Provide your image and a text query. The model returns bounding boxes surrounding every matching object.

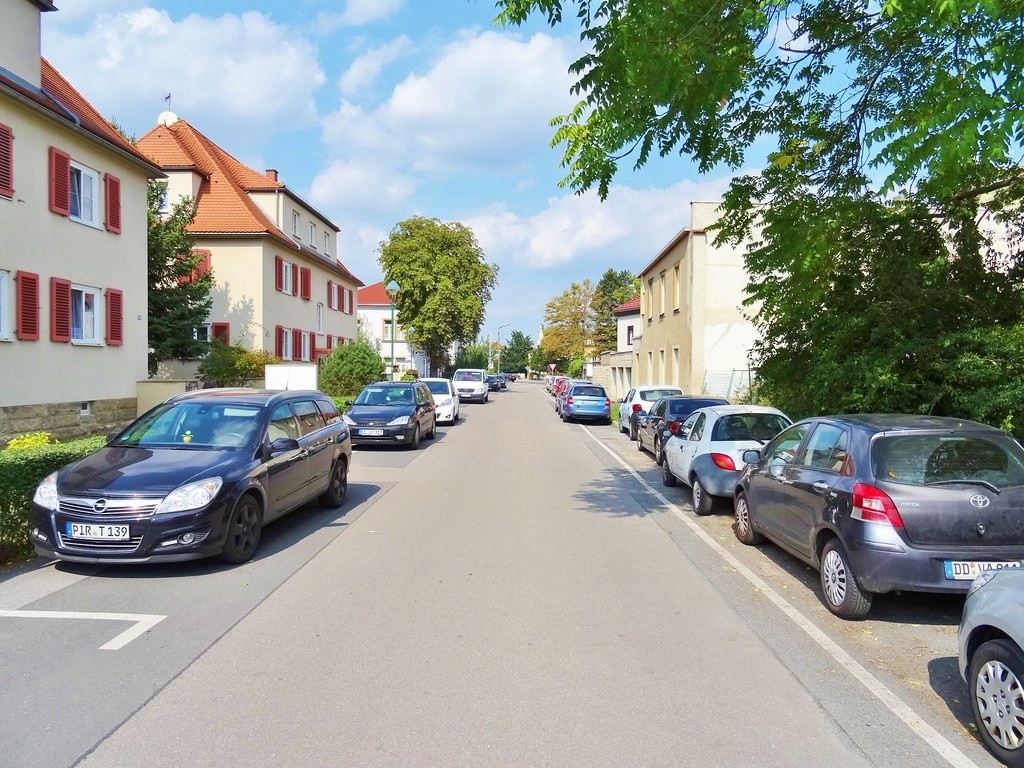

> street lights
[385,276,401,380]
[408,325,416,376]
[496,323,510,374]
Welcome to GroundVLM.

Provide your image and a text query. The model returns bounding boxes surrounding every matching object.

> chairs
[726,418,747,438]
[404,389,412,401]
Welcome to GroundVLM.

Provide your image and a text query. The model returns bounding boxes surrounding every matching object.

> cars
[635,395,732,465]
[545,373,592,413]
[28,384,352,567]
[411,376,460,426]
[957,564,1024,768]
[561,383,612,424]
[486,375,501,392]
[494,374,507,388]
[617,385,685,441]
[662,404,804,516]
[340,378,438,450]
[733,412,1024,621]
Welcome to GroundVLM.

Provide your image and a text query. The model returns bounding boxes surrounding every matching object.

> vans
[452,368,489,404]
